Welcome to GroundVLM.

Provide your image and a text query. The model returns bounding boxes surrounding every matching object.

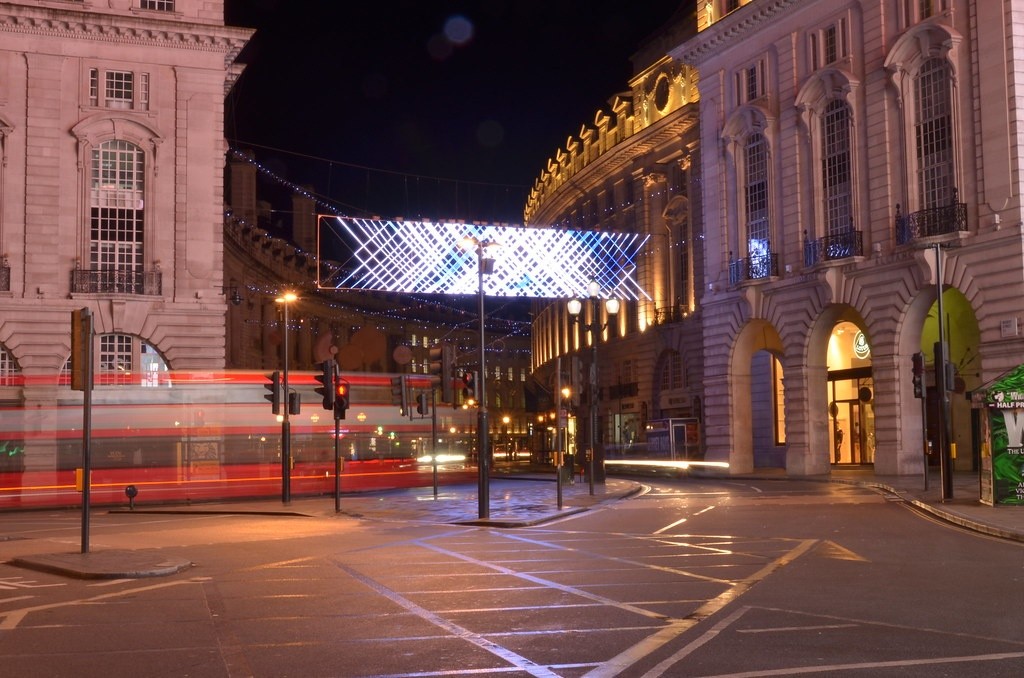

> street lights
[567,271,622,486]
[458,237,505,520]
[281,289,299,506]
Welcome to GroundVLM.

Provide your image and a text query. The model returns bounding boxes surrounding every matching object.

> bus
[0,366,478,508]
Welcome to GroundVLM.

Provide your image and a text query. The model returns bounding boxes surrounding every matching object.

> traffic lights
[429,345,452,404]
[263,370,281,415]
[336,378,350,410]
[391,375,409,418]
[416,393,429,416]
[313,360,334,411]
[461,370,479,402]
